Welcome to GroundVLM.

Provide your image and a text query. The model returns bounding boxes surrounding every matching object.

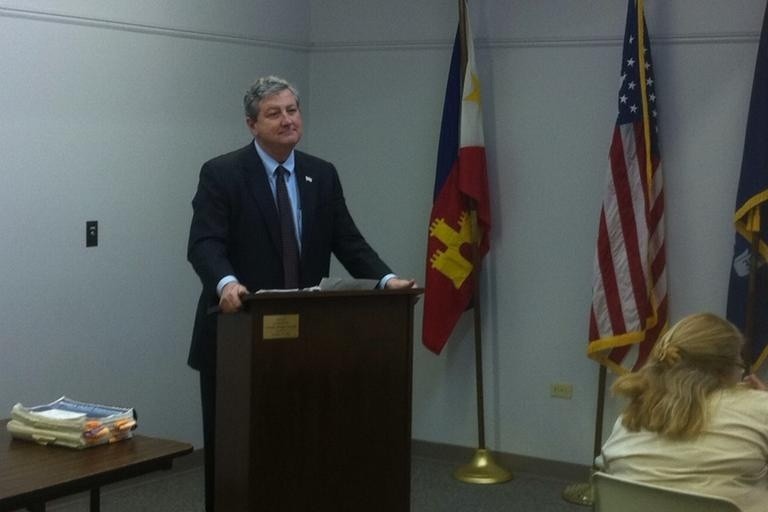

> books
[6,397,135,450]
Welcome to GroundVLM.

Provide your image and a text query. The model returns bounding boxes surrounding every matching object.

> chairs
[588,471,743,512]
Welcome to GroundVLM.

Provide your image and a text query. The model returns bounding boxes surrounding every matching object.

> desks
[0,419,194,512]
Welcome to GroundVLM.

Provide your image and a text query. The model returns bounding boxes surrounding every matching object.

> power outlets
[548,383,573,399]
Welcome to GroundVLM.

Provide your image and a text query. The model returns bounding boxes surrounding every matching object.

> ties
[276,165,301,289]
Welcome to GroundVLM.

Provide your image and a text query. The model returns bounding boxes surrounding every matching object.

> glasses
[736,361,751,375]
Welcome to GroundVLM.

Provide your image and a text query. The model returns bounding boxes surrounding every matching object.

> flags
[420,0,492,356]
[724,0,767,380]
[586,0,669,376]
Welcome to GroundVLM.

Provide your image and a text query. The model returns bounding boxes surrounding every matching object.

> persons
[592,313,767,512]
[185,75,419,512]
